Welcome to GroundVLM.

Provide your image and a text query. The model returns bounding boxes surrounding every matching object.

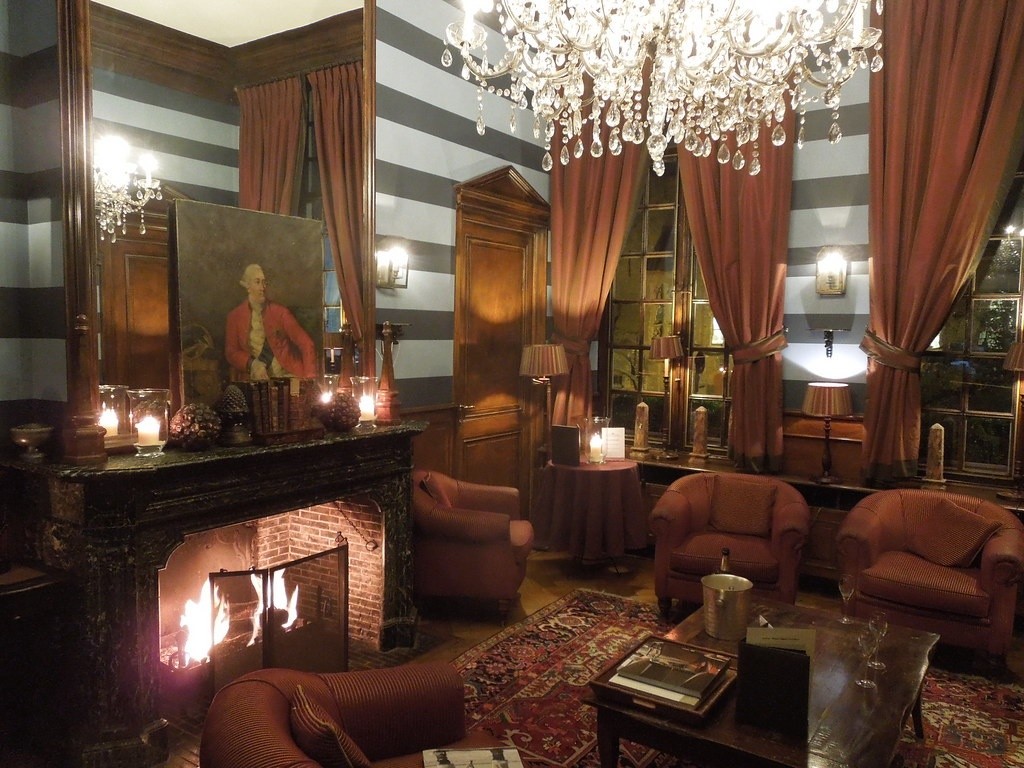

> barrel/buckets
[701,573,754,640]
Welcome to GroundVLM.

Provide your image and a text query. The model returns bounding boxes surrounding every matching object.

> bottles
[719,548,733,574]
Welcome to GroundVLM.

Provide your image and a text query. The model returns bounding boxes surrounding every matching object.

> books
[609,637,732,709]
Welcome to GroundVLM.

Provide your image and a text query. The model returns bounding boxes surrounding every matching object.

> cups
[349,376,378,422]
[323,373,341,398]
[126,387,170,456]
[576,415,612,465]
[98,384,129,437]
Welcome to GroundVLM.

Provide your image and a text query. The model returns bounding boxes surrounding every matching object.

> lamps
[802,382,854,485]
[649,335,685,461]
[93,134,163,244]
[438,0,887,175]
[374,235,413,288]
[815,246,849,295]
[518,345,569,453]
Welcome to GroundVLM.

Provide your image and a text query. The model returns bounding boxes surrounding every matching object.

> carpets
[445,586,1024,768]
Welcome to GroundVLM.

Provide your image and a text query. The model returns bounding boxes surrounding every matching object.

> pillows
[903,498,1002,567]
[705,474,777,539]
[289,683,372,768]
[421,472,451,506]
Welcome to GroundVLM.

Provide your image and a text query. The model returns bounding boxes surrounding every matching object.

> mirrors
[51,0,377,451]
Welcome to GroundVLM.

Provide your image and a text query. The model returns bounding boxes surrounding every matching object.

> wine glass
[838,574,856,624]
[868,611,888,670]
[856,629,876,688]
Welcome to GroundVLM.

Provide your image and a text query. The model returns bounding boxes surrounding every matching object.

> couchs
[412,469,535,620]
[198,667,533,768]
[648,472,811,616]
[834,488,1024,675]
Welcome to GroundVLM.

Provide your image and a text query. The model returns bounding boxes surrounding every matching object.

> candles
[126,389,169,458]
[350,377,379,429]
[325,374,340,402]
[584,417,611,464]
[99,385,128,436]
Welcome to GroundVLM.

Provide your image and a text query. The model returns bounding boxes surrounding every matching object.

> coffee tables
[579,594,942,768]
[535,459,648,576]
